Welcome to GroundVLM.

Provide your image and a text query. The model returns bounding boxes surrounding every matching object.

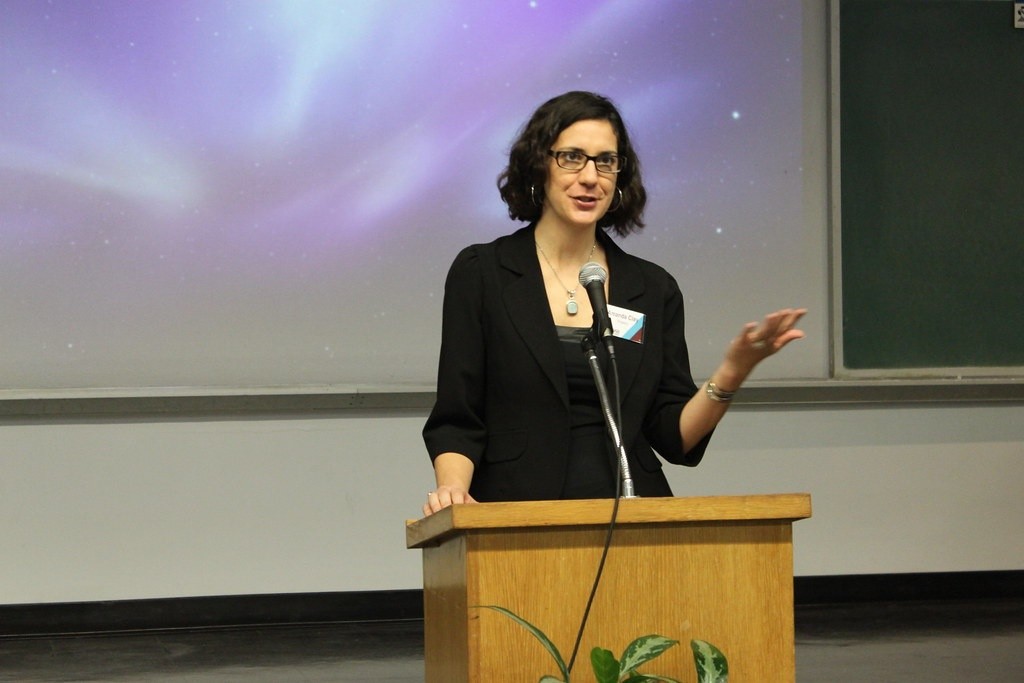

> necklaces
[533,240,598,314]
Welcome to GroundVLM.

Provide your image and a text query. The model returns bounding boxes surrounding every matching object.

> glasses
[549,149,627,174]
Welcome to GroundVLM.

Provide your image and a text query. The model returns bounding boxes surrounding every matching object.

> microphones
[579,262,616,360]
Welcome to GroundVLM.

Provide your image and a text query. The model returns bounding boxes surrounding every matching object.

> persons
[421,91,808,518]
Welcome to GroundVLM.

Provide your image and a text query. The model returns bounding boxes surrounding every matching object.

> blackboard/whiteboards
[826,0,1024,379]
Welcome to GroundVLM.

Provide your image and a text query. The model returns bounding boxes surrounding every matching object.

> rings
[752,340,768,348]
[429,492,432,496]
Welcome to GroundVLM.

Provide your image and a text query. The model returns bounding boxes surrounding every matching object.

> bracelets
[706,377,736,401]
